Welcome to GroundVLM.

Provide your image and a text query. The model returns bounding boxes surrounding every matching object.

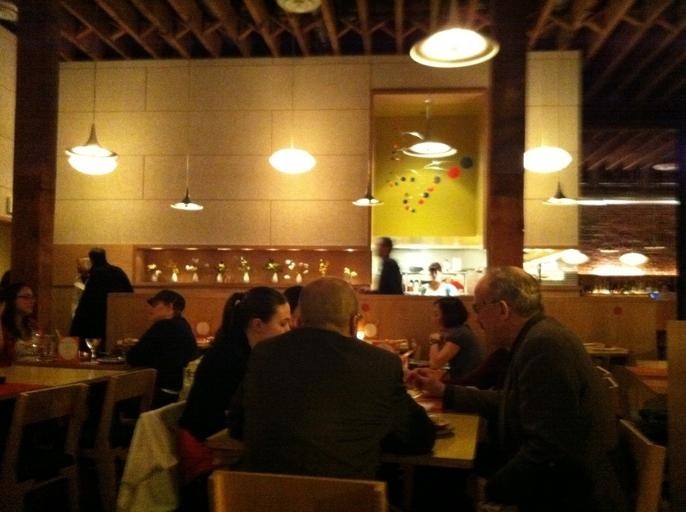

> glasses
[18,295,35,300]
[472,299,513,314]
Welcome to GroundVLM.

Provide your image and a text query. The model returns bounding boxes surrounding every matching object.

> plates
[98,356,127,364]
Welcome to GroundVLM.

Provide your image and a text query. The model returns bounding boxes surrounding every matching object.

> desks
[204,389,479,472]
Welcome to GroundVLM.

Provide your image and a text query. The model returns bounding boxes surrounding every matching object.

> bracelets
[429,339,442,347]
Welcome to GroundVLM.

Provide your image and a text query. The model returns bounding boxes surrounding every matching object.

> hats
[148,291,185,310]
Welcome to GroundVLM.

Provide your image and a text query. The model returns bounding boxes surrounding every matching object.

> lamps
[403,99,459,158]
[268,57,316,174]
[541,97,571,205]
[63,39,118,176]
[352,98,382,207]
[169,52,203,211]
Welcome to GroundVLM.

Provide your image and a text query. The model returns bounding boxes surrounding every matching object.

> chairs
[366,337,450,373]
[616,418,665,511]
[139,401,188,511]
[2,342,158,511]
[210,469,387,512]
[181,335,212,400]
[582,337,669,418]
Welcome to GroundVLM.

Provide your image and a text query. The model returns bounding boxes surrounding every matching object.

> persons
[359,237,404,294]
[0,283,43,368]
[418,262,459,296]
[428,297,479,379]
[407,266,634,512]
[70,249,134,352]
[180,285,307,480]
[81,289,200,450]
[227,276,436,481]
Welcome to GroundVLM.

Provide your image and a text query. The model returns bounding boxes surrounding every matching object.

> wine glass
[84,337,101,364]
